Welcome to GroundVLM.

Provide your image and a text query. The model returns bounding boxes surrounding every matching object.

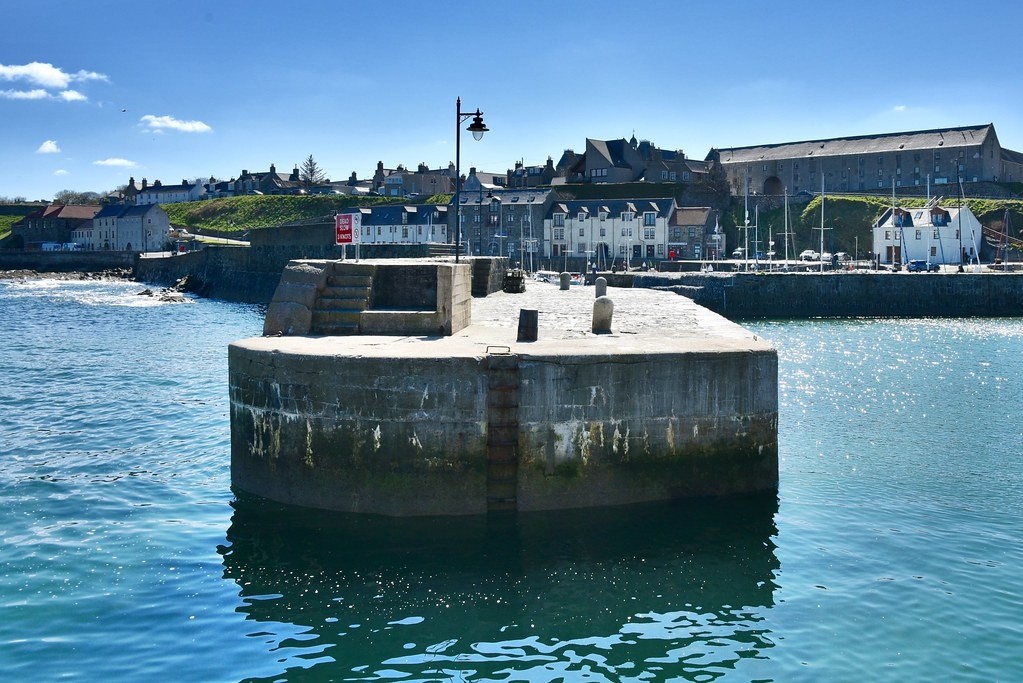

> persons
[668,248,678,263]
[570,275,581,282]
[833,254,840,274]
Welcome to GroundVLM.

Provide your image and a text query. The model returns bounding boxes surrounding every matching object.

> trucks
[41,244,62,252]
[732,248,750,260]
[62,242,83,252]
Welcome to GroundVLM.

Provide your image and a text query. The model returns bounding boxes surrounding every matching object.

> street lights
[455,96,490,264]
[479,182,493,256]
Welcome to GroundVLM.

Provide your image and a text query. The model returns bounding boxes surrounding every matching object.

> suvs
[799,249,815,261]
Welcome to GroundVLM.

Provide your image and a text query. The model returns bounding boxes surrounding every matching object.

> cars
[811,253,821,261]
[821,252,833,262]
[834,252,851,261]
[908,259,940,273]
[753,250,767,260]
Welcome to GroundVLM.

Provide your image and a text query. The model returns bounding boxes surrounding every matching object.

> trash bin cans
[505,270,522,293]
